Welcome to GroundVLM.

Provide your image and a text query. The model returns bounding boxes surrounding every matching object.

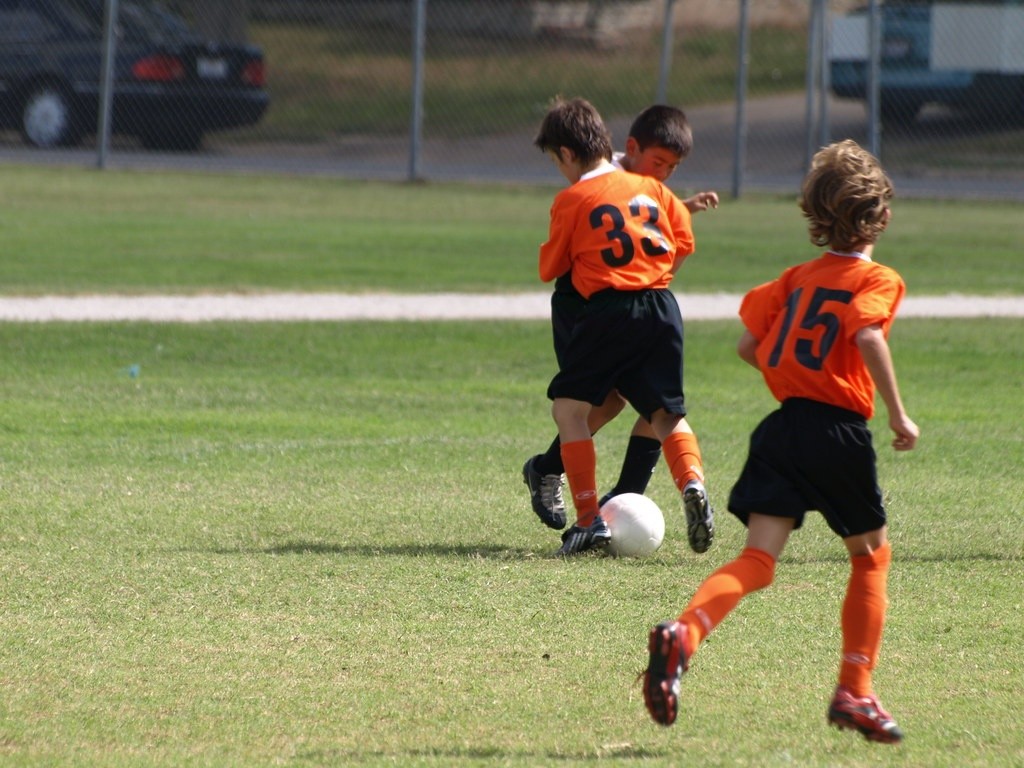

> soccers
[600,492,665,559]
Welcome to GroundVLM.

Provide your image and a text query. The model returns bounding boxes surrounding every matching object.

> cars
[0,1,275,153]
[826,1,1022,125]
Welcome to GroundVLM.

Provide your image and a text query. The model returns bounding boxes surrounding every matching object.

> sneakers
[681,486,716,557]
[824,688,903,746]
[551,517,613,557]
[642,618,694,726]
[521,452,568,530]
[594,493,615,508]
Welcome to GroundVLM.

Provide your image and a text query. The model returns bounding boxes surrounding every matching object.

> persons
[523,95,719,558]
[642,140,919,744]
